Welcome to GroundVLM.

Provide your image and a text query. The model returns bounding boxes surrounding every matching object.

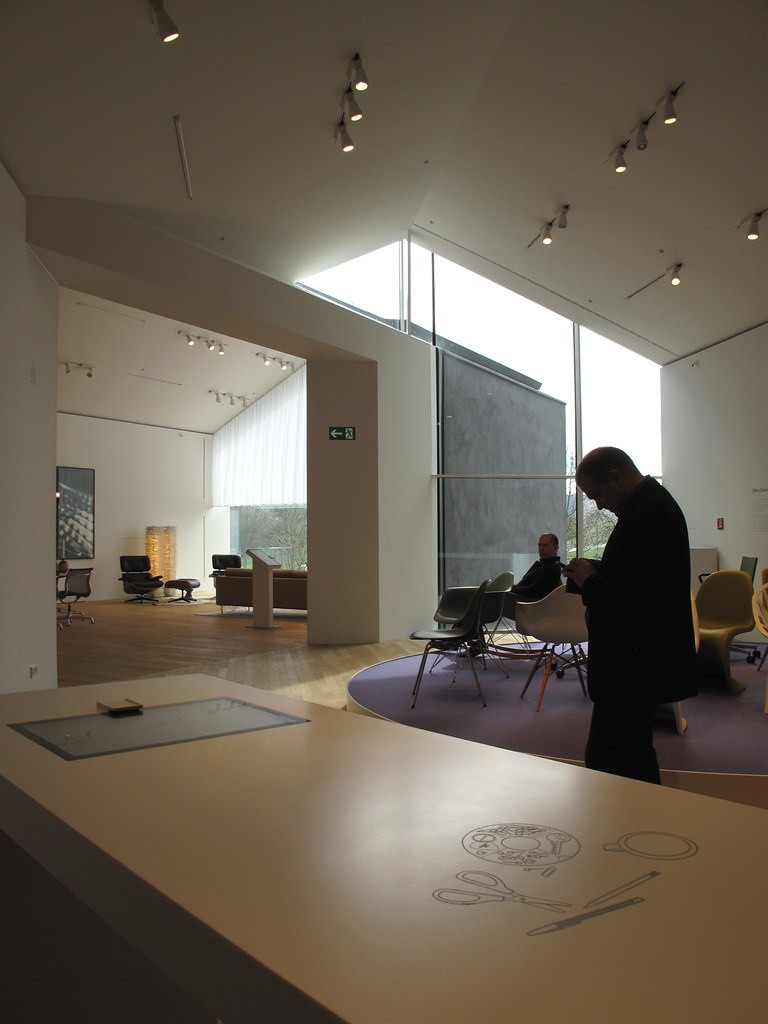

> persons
[457,533,561,658]
[562,446,698,784]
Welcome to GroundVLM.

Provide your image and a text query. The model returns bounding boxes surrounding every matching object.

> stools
[167,578,200,603]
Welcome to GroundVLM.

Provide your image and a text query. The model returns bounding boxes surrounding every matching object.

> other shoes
[432,641,459,651]
[458,639,486,658]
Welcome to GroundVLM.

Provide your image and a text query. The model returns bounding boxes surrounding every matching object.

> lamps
[349,53,368,91]
[343,81,363,121]
[337,120,355,153]
[737,208,768,241]
[667,262,683,286]
[525,80,684,252]
[174,113,193,201]
[145,0,180,44]
[64,330,297,408]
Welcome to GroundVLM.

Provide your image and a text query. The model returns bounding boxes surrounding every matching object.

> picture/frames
[55,466,95,560]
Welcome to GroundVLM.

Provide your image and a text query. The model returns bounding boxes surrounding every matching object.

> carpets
[195,607,308,622]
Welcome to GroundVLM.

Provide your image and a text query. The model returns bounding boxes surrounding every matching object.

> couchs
[216,566,308,614]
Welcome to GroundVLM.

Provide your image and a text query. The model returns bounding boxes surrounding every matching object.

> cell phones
[555,561,567,567]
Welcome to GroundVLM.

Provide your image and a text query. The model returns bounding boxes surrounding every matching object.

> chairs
[407,556,768,734]
[56,568,94,628]
[118,555,163,604]
[210,554,242,589]
